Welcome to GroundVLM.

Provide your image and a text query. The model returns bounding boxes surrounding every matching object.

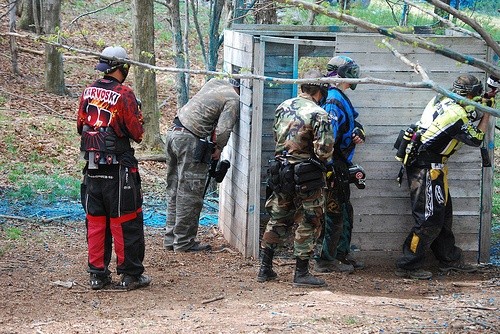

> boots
[293,256,325,288]
[257,246,277,282]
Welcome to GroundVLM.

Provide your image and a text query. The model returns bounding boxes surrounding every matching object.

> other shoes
[128,275,150,290]
[90,273,112,290]
[313,260,353,274]
[345,255,365,268]
[439,262,477,272]
[178,243,212,254]
[395,267,432,279]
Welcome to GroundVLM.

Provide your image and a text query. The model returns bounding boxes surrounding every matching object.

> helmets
[454,74,478,94]
[326,55,359,90]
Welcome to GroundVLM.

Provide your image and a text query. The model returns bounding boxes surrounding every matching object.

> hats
[94,46,127,72]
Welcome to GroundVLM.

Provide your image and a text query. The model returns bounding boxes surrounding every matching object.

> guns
[469,74,500,122]
[201,150,231,200]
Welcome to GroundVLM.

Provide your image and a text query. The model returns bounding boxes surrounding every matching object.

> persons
[163,66,241,251]
[256,70,334,288]
[395,73,496,279]
[78,45,152,290]
[315,55,365,274]
